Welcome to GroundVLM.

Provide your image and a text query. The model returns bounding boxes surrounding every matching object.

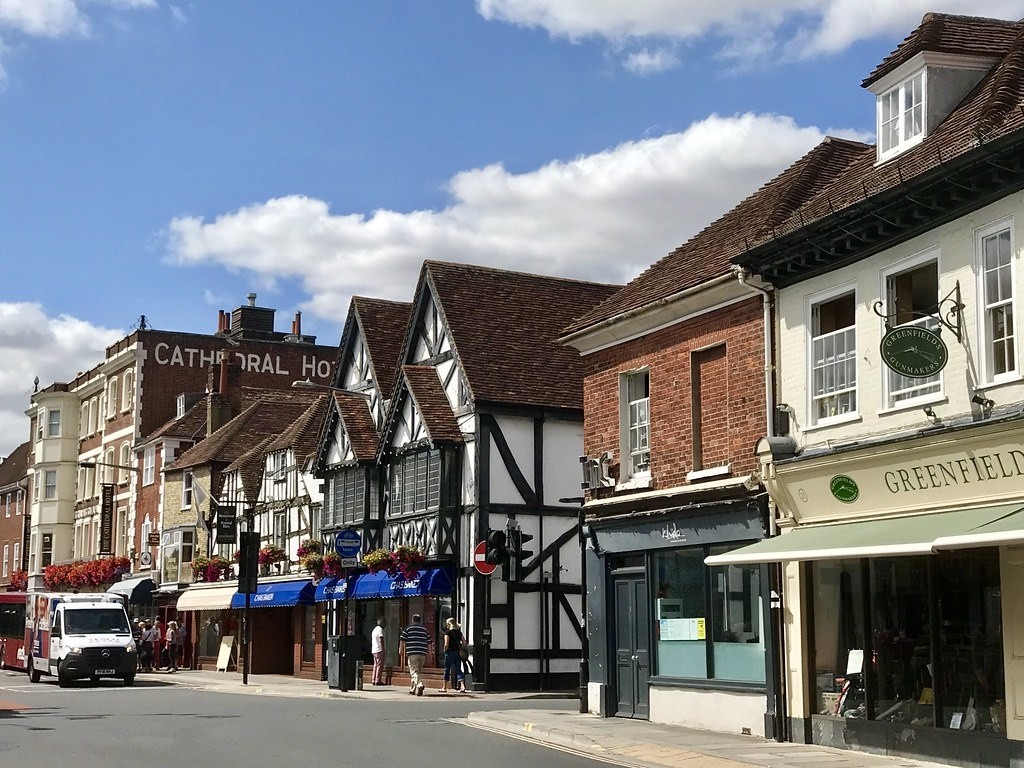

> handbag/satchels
[175,647,183,656]
[138,651,147,661]
[459,646,469,661]
[161,647,169,657]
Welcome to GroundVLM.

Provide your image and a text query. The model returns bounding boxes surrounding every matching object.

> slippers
[438,689,446,692]
[456,689,467,693]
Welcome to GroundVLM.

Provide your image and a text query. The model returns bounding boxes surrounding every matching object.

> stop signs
[473,541,500,575]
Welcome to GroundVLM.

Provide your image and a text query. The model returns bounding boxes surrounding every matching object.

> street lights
[318,524,344,682]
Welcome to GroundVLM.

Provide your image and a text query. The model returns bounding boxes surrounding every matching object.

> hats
[145,618,150,622]
[134,617,140,623]
[138,622,146,628]
[167,621,175,625]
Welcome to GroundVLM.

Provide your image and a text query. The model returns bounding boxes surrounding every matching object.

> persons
[30,596,43,658]
[130,615,186,673]
[371,617,387,685]
[398,614,433,696]
[437,617,467,694]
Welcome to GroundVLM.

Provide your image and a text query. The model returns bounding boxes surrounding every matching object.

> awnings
[106,577,156,605]
[175,587,238,611]
[703,500,1024,565]
[314,569,452,602]
[231,581,315,609]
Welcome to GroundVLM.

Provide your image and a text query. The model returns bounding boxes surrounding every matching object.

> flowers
[362,547,425,583]
[295,540,345,582]
[191,555,230,583]
[44,556,132,592]
[11,566,28,592]
[233,544,288,566]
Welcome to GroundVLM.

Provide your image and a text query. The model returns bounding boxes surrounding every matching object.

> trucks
[24,592,138,689]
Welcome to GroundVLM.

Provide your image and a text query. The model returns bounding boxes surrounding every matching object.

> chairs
[98,614,113,630]
[957,650,993,711]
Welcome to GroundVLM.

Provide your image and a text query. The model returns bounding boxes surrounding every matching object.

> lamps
[77,462,142,473]
[292,377,372,402]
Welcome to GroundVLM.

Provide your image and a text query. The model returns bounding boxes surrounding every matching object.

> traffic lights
[518,531,534,580]
[484,528,509,565]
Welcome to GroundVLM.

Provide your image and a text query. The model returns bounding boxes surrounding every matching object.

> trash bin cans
[327,635,361,689]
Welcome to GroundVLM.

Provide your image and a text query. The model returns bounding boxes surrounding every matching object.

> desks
[913,644,980,709]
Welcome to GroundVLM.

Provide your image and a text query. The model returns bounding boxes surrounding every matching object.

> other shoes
[408,691,415,695]
[373,682,384,685]
[168,667,176,673]
[136,667,153,673]
[416,685,425,696]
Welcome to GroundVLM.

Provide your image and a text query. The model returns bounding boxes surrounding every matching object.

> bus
[0,592,27,671]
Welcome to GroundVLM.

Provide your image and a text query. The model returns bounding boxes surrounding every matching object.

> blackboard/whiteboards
[216,635,238,672]
